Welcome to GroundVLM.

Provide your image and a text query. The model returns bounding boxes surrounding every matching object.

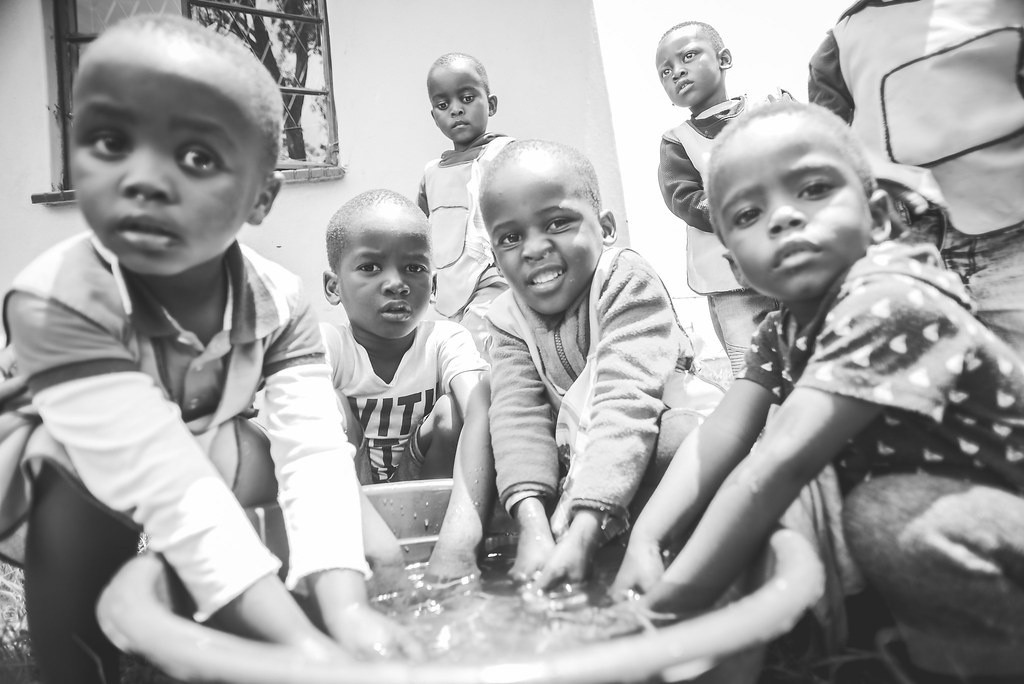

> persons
[0,12,421,684]
[311,0,1024,684]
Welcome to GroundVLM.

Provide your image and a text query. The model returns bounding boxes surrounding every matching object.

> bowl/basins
[101,478,829,684]
